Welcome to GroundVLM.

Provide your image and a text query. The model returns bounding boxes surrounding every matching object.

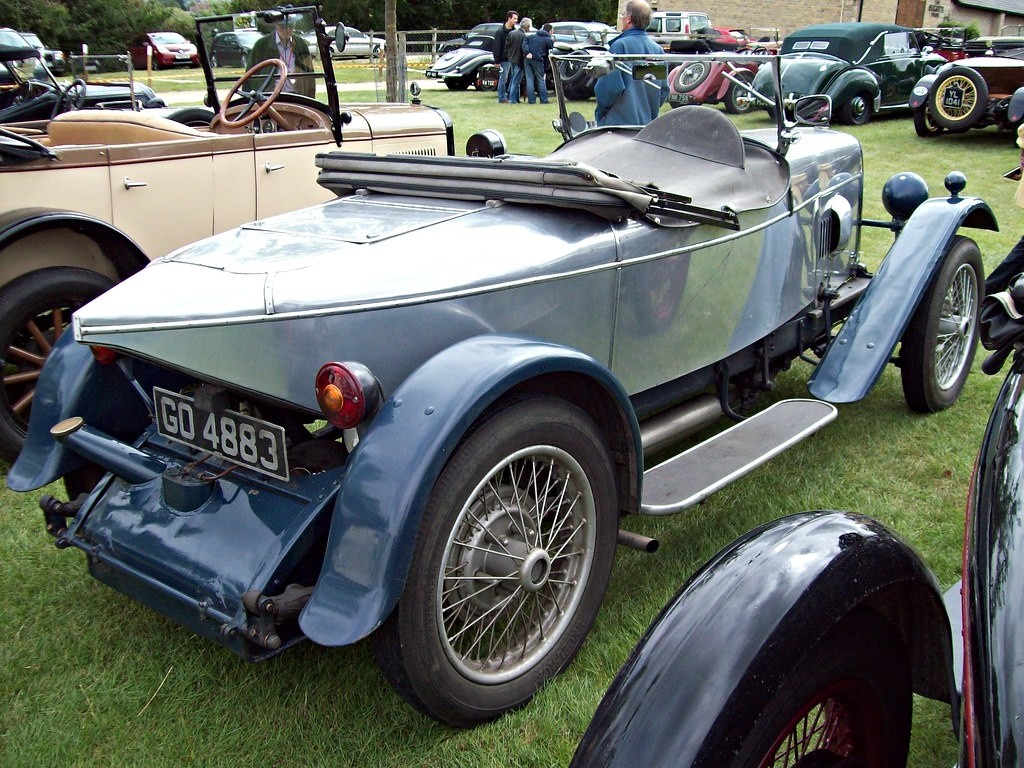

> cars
[18,33,66,77]
[0,27,47,81]
[209,30,263,69]
[438,22,540,54]
[563,368,1024,768]
[297,25,386,61]
[130,32,200,71]
[424,34,500,92]
[540,21,620,45]
[544,12,1024,139]
[6,26,1003,735]
[0,23,455,469]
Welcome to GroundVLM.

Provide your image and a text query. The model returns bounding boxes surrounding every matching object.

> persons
[594,0,671,126]
[493,11,556,103]
[242,13,315,98]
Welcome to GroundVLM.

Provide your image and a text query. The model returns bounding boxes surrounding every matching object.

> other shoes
[499,99,508,103]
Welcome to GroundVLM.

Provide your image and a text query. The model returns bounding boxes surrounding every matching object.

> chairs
[47,109,232,146]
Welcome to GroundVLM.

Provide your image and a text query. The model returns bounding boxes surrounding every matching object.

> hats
[276,13,303,24]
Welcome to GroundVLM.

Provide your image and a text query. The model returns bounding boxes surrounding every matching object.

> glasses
[620,13,630,17]
